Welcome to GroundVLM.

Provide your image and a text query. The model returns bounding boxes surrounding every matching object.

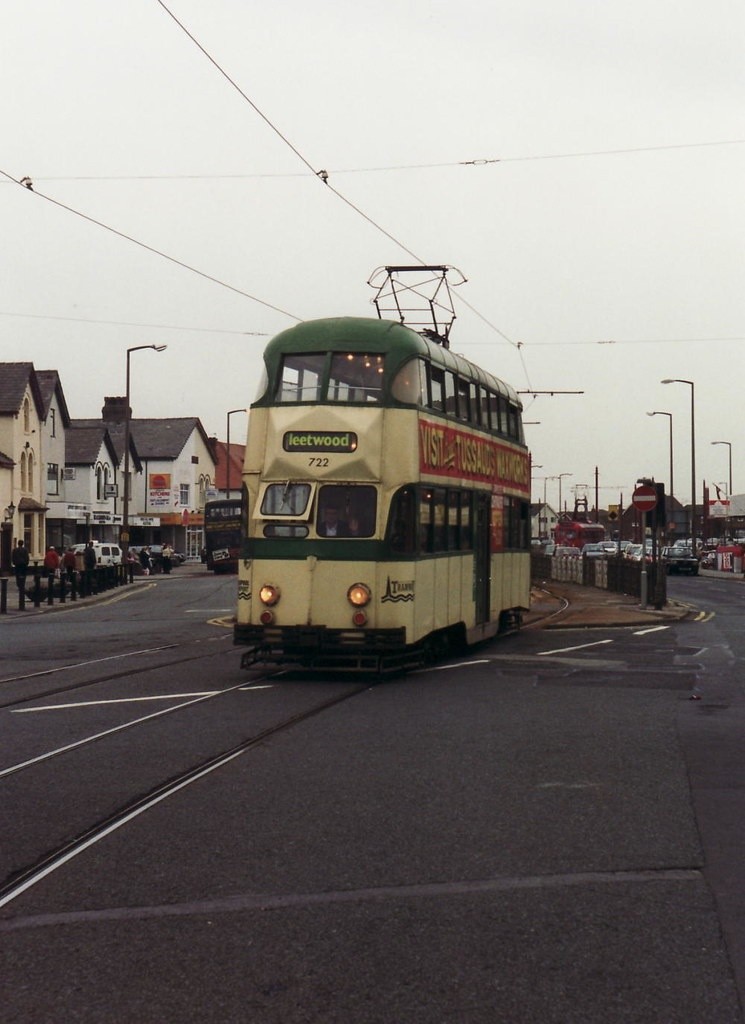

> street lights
[661,379,697,557]
[121,345,167,563]
[647,410,675,546]
[711,441,732,494]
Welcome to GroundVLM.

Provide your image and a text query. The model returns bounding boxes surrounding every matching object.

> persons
[127,542,172,576]
[12,540,33,602]
[317,503,352,537]
[84,541,96,570]
[44,545,78,586]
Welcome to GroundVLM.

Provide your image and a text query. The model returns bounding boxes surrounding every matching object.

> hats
[50,546,54,548]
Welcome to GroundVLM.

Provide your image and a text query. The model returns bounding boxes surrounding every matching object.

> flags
[716,487,721,500]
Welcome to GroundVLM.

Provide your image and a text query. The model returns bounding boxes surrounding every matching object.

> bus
[232,317,532,674]
[204,499,242,574]
[554,521,605,549]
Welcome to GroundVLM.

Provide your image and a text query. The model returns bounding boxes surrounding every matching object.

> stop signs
[632,486,658,511]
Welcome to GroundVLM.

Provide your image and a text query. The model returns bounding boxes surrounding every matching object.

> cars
[531,539,701,577]
[149,544,186,564]
[672,534,745,549]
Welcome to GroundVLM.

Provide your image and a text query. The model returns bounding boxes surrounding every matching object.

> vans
[72,542,123,568]
